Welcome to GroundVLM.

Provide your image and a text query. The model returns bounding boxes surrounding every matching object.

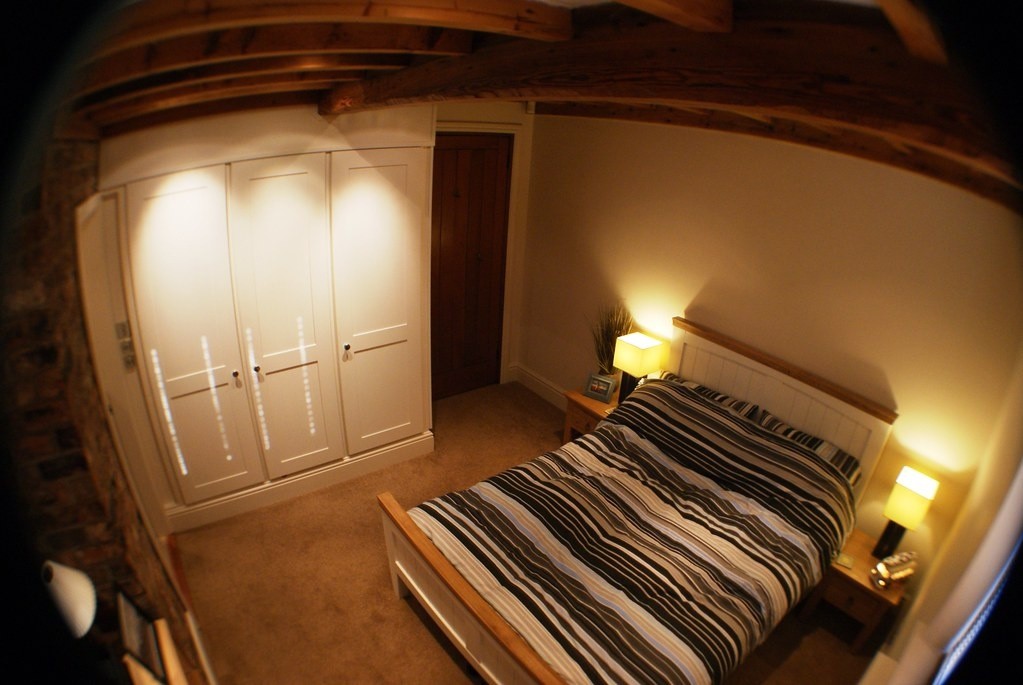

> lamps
[871,465,940,560]
[612,332,671,403]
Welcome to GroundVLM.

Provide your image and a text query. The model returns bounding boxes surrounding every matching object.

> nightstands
[560,387,620,447]
[796,528,905,656]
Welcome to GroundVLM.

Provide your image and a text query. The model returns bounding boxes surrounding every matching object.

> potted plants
[585,299,636,392]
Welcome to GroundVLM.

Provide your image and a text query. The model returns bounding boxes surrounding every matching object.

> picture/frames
[582,373,616,404]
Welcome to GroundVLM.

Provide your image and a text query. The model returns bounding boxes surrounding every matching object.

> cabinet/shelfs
[83,96,433,535]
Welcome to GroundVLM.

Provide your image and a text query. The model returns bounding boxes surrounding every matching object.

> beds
[378,315,900,685]
